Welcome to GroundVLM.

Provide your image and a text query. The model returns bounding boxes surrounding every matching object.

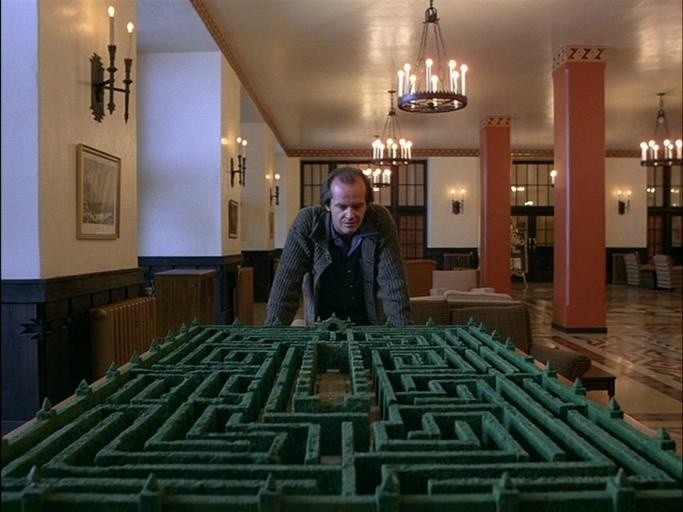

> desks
[581,366,616,400]
[154,269,216,334]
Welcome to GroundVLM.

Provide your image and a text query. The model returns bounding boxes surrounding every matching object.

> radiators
[90,297,157,378]
[612,254,625,283]
[237,267,253,325]
[443,253,471,270]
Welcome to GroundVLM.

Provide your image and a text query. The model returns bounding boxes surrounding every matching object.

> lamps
[270,173,281,206]
[397,0,468,113]
[451,188,465,214]
[617,190,632,214]
[230,137,248,187]
[371,90,413,166]
[362,135,392,188]
[89,6,134,123]
[640,92,683,167]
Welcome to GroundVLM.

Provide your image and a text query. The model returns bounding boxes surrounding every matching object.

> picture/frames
[229,199,239,238]
[75,142,122,239]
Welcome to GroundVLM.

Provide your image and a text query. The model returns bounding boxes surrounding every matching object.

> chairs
[402,260,591,382]
[624,253,656,288]
[653,254,683,292]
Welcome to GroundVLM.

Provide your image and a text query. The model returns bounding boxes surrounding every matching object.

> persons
[263,166,414,328]
